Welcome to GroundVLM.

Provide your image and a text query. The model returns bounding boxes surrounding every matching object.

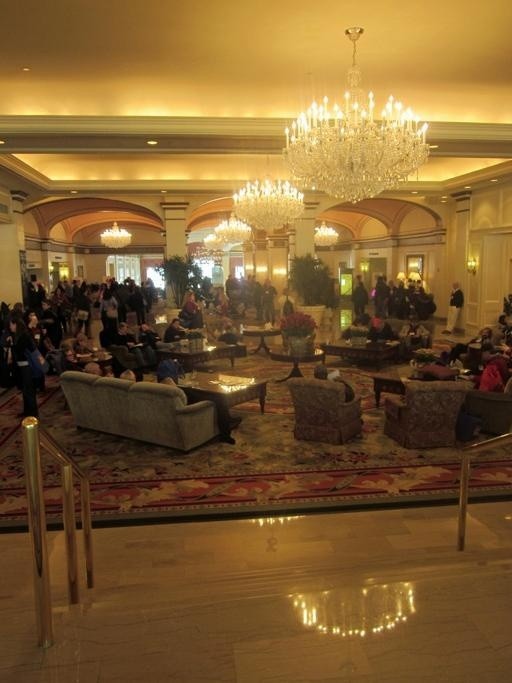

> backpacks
[158,358,179,386]
[420,364,460,381]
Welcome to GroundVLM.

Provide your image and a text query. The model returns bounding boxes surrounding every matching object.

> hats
[372,318,383,334]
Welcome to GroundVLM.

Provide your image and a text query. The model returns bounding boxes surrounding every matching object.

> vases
[284,332,315,353]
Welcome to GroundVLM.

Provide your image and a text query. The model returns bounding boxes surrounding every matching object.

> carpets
[0,339,512,532]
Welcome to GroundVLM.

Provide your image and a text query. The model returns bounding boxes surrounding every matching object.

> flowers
[280,312,318,336]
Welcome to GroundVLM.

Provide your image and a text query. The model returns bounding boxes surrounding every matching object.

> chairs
[384,381,468,449]
[461,377,512,436]
[288,377,361,444]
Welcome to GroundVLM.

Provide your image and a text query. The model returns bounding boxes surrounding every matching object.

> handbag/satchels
[25,348,49,374]
[77,310,89,321]
[479,364,504,394]
[106,307,118,318]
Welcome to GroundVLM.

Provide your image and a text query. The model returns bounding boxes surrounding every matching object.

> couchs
[343,319,434,361]
[60,371,220,455]
[112,320,225,367]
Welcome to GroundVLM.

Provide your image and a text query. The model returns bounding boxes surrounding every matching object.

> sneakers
[219,435,235,445]
[441,329,453,335]
[228,416,241,428]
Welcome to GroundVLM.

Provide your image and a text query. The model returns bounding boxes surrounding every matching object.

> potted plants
[155,255,201,323]
[290,255,333,332]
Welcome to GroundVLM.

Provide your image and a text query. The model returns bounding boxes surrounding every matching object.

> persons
[315,364,355,403]
[353,275,426,321]
[1,271,280,446]
[439,293,512,445]
[339,312,394,342]
[440,282,464,334]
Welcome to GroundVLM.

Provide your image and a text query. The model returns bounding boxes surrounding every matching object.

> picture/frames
[405,255,424,282]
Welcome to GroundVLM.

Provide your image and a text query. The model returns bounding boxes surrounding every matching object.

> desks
[244,327,280,354]
[154,338,414,415]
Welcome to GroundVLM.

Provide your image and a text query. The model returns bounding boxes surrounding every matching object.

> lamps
[100,221,131,249]
[396,271,422,287]
[314,221,339,247]
[284,29,430,202]
[467,255,476,275]
[204,153,304,251]
